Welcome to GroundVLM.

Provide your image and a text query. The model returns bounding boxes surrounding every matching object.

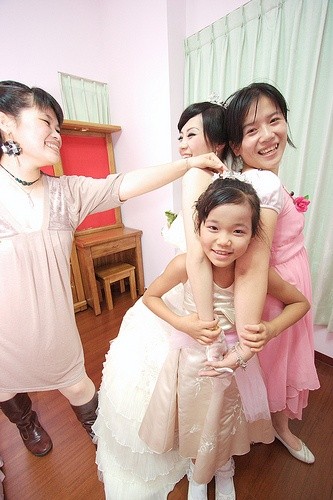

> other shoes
[215,476,236,500]
[272,425,315,464]
[188,481,208,500]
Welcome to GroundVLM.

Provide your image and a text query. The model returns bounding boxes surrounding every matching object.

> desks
[75,223,145,316]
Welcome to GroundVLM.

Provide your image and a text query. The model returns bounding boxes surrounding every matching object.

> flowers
[290,192,311,216]
[160,208,178,242]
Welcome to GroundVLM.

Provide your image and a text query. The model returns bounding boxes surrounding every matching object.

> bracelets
[231,346,247,373]
[211,325,219,331]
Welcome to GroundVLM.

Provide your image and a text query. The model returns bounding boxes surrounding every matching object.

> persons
[93,82,317,500]
[0,82,236,457]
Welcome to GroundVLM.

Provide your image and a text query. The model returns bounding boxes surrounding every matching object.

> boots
[71,389,101,449]
[0,392,52,456]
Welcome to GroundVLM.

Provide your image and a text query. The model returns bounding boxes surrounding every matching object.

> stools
[96,262,137,307]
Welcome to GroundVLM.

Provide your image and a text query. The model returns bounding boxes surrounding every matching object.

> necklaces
[0,163,44,186]
